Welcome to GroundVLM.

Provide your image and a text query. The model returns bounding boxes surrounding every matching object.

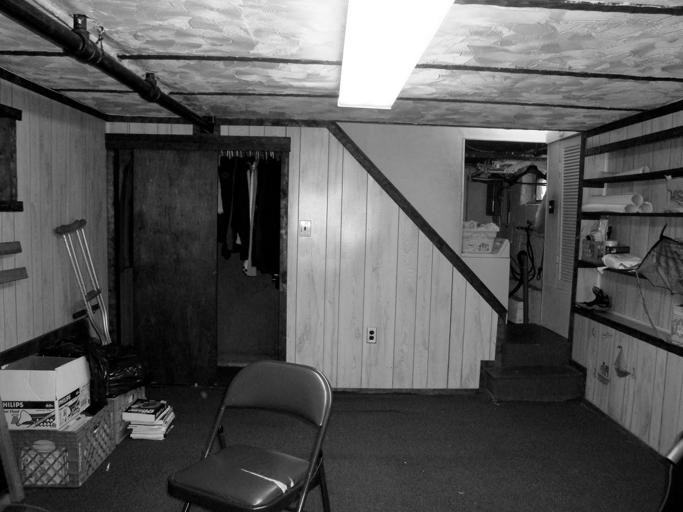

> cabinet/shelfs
[567,100,683,475]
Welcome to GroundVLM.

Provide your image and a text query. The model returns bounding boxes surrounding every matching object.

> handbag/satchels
[29,332,147,419]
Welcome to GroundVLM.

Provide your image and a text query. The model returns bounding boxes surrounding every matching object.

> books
[121,398,176,440]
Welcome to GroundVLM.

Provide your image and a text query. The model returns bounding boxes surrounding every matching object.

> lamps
[336,0,454,109]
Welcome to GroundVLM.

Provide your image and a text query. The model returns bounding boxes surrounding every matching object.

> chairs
[166,359,334,512]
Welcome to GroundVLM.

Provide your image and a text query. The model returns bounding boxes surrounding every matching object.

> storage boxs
[0,353,91,432]
[462,227,500,253]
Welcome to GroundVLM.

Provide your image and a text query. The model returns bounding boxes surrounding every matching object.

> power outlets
[368,327,376,343]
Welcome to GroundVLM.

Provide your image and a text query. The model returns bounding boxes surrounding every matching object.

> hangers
[470,149,548,190]
[216,148,277,171]
